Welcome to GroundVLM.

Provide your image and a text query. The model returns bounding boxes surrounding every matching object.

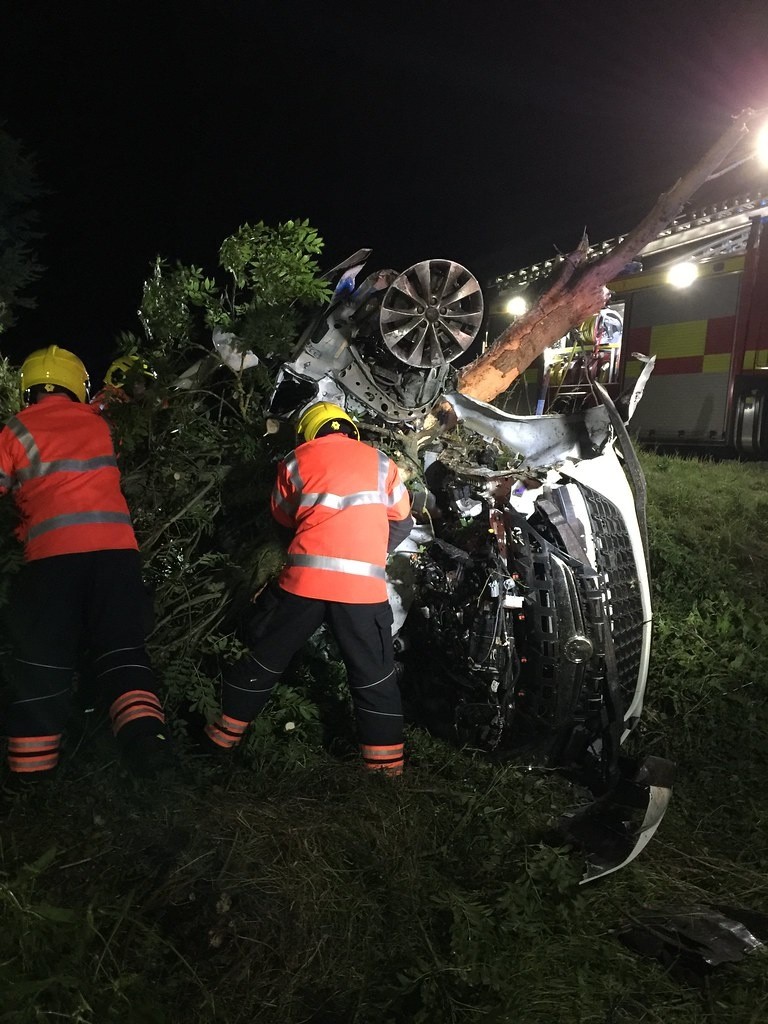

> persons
[191,401,414,783]
[88,353,170,467]
[1,345,181,787]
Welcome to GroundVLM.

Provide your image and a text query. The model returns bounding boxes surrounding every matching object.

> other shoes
[0,770,55,801]
[125,730,175,775]
[176,697,232,766]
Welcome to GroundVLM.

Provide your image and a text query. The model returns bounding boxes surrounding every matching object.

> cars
[274,247,664,755]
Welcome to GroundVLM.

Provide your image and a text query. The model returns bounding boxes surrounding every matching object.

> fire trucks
[472,192,767,452]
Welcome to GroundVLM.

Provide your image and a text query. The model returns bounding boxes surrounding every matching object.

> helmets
[103,355,158,389]
[17,345,89,404]
[298,401,361,442]
